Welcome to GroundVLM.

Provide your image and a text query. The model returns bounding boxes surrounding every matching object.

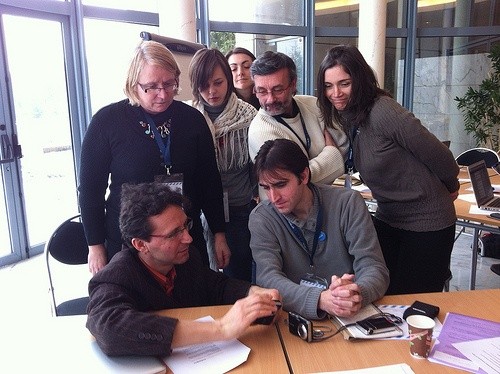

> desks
[275,289,500,374]
[333,166,500,291]
[152,304,290,374]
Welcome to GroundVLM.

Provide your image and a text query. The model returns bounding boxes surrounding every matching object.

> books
[91,337,167,374]
[335,303,405,338]
[427,310,500,374]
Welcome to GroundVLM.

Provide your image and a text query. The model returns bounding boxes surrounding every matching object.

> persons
[316,44,460,295]
[226,47,262,113]
[86,181,284,360]
[247,51,350,204]
[182,48,259,276]
[248,139,390,320]
[77,44,231,307]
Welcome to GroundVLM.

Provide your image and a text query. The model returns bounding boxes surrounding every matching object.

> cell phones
[357,317,396,334]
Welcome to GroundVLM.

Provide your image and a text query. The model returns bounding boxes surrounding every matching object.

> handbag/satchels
[225,199,259,253]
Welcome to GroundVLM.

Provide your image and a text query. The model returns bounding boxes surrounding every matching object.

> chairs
[454,147,500,168]
[44,213,89,317]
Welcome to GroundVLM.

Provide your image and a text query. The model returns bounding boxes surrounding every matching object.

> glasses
[137,82,176,94]
[146,216,194,242]
[253,82,291,97]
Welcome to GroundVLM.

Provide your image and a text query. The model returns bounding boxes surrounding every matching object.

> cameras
[254,299,282,326]
[287,311,313,342]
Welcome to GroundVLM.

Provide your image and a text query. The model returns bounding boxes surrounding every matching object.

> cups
[406,314,436,359]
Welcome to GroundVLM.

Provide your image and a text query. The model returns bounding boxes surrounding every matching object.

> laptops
[468,159,500,212]
